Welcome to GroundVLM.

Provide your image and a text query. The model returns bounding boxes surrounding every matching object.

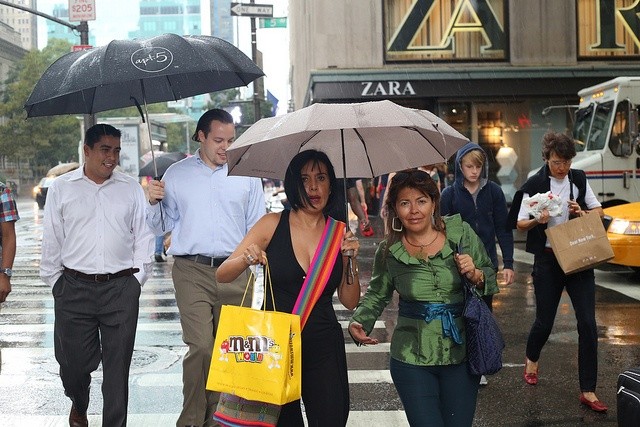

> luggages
[616,368,640,426]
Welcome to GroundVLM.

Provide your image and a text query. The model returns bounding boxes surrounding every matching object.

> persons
[349,170,499,427]
[0,182,20,302]
[517,131,608,413]
[329,172,369,236]
[41,122,156,427]
[379,169,397,235]
[215,149,361,427]
[355,175,368,211]
[146,108,268,426]
[438,142,514,315]
[147,180,169,263]
[418,162,441,227]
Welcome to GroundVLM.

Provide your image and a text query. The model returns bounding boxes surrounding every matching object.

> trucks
[527,76,640,212]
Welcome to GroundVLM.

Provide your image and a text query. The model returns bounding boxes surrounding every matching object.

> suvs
[32,163,80,210]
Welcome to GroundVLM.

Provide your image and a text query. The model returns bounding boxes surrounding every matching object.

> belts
[180,255,228,266]
[62,265,140,283]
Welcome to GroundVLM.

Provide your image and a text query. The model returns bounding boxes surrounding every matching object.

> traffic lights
[221,104,243,125]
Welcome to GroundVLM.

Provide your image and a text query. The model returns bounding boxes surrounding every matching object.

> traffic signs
[230,2,273,17]
[259,16,288,29]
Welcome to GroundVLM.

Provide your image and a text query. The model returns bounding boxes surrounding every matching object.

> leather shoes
[579,391,608,411]
[69,402,88,426]
[524,355,539,385]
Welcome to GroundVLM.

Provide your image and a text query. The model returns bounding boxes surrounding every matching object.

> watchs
[0,269,12,277]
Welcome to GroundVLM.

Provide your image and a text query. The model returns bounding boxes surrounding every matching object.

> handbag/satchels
[544,202,615,275]
[454,243,507,376]
[205,254,304,406]
[210,393,283,424]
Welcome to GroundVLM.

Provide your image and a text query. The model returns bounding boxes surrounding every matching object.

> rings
[247,255,254,262]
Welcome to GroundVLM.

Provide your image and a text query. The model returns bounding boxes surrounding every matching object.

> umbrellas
[225,99,470,257]
[138,152,187,177]
[24,33,267,202]
[45,159,79,176]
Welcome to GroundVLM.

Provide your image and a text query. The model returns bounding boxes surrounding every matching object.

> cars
[602,201,640,267]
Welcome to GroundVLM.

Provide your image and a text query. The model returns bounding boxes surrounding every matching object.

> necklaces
[403,231,439,261]
